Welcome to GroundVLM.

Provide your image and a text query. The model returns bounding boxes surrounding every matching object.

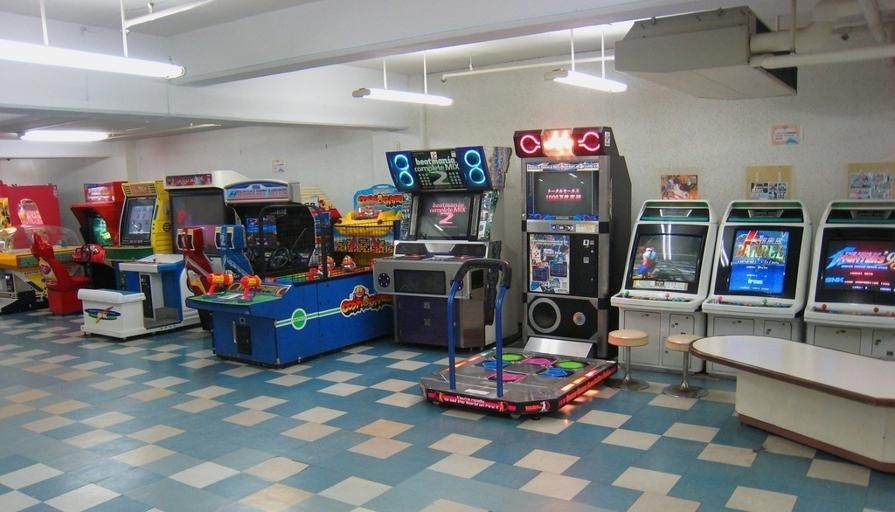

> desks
[689,335,895,474]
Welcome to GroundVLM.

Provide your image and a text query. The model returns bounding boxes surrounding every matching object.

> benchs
[78,288,147,337]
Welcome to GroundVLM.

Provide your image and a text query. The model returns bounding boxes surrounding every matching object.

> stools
[608,329,650,392]
[662,334,710,400]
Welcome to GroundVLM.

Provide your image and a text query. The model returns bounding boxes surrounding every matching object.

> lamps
[352,52,456,108]
[544,28,628,93]
[0,0,185,80]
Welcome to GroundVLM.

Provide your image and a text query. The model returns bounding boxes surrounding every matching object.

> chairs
[213,224,254,289]
[175,226,222,296]
[30,231,92,317]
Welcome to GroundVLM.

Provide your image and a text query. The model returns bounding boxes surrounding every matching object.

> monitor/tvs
[815,226,894,308]
[526,160,600,221]
[625,224,709,294]
[84,211,113,247]
[714,225,804,300]
[169,189,224,257]
[245,215,279,251]
[410,192,482,241]
[120,195,157,245]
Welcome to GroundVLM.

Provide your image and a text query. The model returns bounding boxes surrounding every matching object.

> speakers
[522,293,604,342]
